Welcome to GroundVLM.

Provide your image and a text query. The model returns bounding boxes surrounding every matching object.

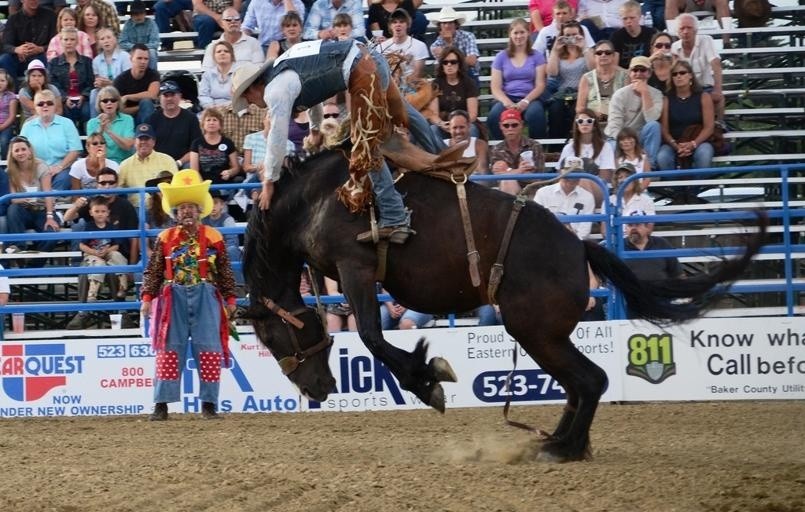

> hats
[209,189,230,202]
[28,59,46,70]
[230,57,276,112]
[145,171,173,194]
[424,7,466,24]
[391,8,410,19]
[161,81,179,95]
[560,156,585,170]
[630,56,651,69]
[135,124,154,139]
[500,109,521,121]
[127,0,146,14]
[158,169,214,220]
[615,163,636,175]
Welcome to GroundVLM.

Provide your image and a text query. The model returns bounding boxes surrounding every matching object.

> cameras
[563,36,579,45]
[662,53,675,61]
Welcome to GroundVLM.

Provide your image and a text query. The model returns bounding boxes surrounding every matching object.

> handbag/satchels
[679,126,726,151]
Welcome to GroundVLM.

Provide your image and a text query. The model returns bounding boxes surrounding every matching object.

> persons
[139,200,240,419]
[2,0,776,337]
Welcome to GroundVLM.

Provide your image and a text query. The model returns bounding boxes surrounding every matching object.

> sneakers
[121,313,133,328]
[202,403,224,420]
[150,403,167,421]
[117,289,126,298]
[68,313,96,330]
[88,296,96,302]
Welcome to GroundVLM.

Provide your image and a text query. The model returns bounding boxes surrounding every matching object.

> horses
[228,138,767,464]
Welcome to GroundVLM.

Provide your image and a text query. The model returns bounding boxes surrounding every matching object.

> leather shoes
[357,225,408,244]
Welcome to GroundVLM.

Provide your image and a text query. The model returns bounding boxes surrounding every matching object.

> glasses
[633,67,647,72]
[594,50,614,55]
[672,71,691,77]
[100,98,118,103]
[654,43,671,49]
[575,119,595,124]
[324,113,339,119]
[503,123,520,128]
[563,20,579,25]
[37,101,54,106]
[97,180,116,185]
[11,136,27,142]
[441,60,459,65]
[222,16,241,21]
[91,141,106,145]
